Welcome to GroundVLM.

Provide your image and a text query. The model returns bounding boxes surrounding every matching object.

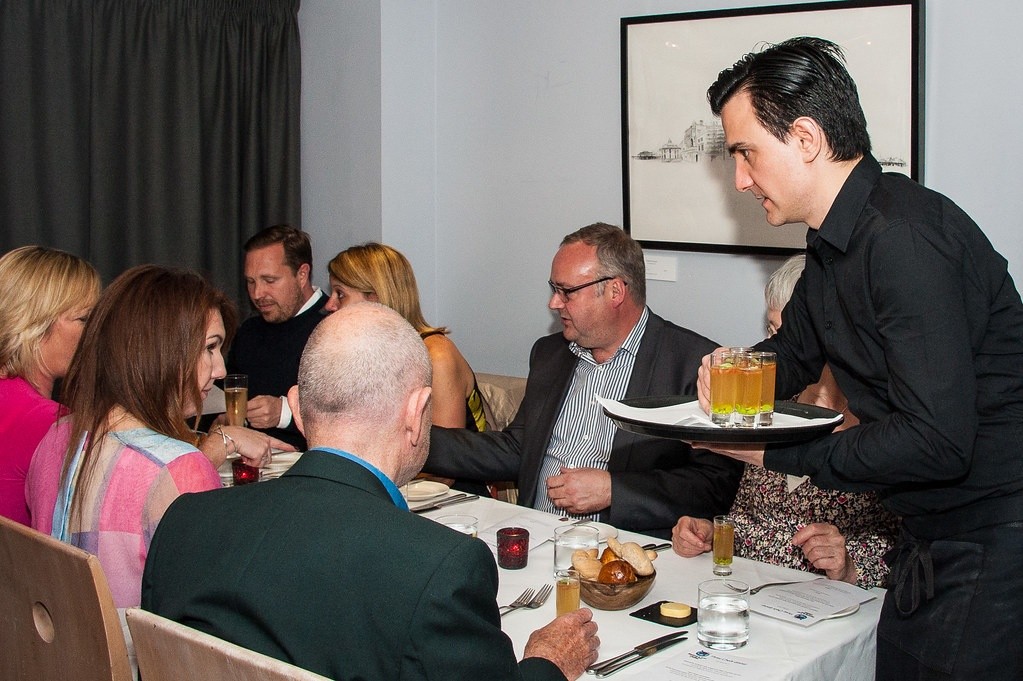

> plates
[407,481,449,501]
[548,520,618,543]
[193,429,304,488]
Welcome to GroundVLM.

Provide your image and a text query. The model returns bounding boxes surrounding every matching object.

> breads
[569,537,658,586]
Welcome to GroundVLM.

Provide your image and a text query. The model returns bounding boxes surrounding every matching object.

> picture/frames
[621,0,927,256]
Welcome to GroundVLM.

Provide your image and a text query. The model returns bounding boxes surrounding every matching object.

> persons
[672,255,906,590]
[186,226,330,454]
[137,300,601,681]
[26,262,237,680]
[681,37,1023,681]
[0,246,300,528]
[325,241,502,501]
[420,224,744,542]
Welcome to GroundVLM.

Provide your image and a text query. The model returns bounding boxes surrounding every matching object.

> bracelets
[210,425,238,456]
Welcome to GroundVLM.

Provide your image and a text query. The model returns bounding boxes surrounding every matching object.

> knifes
[586,630,689,675]
[597,637,689,679]
[409,493,479,514]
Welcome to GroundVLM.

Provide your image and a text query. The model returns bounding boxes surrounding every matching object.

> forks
[499,588,535,609]
[502,583,554,618]
[724,581,802,595]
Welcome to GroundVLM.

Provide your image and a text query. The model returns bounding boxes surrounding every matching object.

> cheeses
[660,603,691,618]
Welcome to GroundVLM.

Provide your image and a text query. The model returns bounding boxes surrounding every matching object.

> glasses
[548,277,628,303]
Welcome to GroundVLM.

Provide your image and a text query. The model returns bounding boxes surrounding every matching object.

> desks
[188,449,890,681]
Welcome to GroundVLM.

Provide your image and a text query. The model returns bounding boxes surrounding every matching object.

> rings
[262,456,268,460]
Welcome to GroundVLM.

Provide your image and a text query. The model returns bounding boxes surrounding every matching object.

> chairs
[475,372,533,502]
[0,515,130,681]
[125,605,332,681]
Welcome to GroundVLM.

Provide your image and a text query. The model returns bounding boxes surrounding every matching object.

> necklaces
[840,404,848,414]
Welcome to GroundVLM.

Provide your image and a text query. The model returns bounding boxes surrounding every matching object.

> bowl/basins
[567,561,657,611]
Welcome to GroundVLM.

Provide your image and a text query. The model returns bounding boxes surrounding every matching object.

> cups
[223,375,247,427]
[698,579,750,649]
[398,482,407,503]
[555,569,581,617]
[733,352,762,428]
[729,347,754,353]
[497,527,530,569]
[433,514,478,538]
[231,458,260,487]
[713,515,735,565]
[749,350,777,425]
[554,525,599,580]
[710,351,736,425]
